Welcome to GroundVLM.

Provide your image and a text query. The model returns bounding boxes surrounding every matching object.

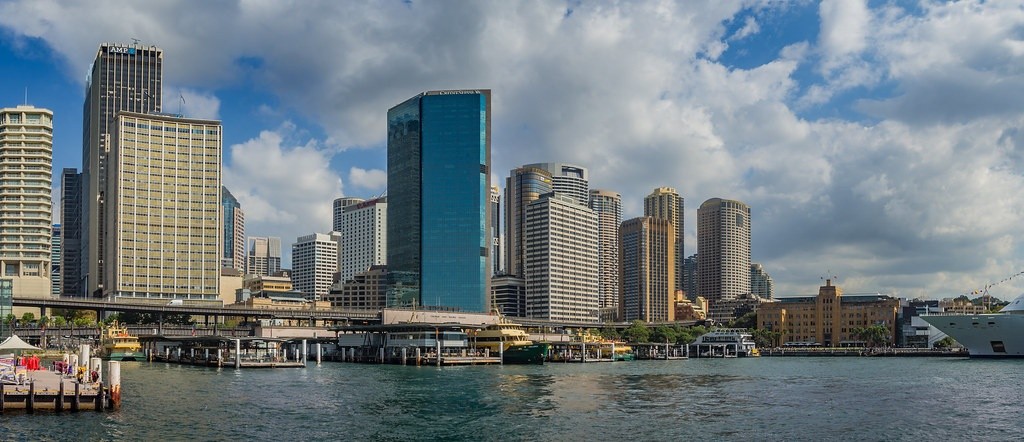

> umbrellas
[0,334,41,380]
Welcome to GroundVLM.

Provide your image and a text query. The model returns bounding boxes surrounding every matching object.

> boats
[90,318,147,362]
[575,327,636,361]
[918,292,1024,359]
[467,314,552,365]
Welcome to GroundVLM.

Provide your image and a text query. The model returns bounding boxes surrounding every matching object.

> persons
[16,354,21,366]
